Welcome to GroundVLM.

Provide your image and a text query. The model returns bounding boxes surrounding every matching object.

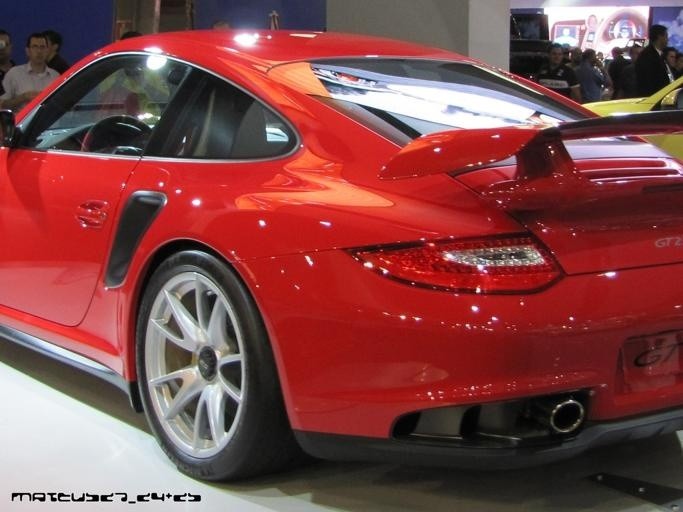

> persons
[99,29,170,127]
[42,30,69,73]
[634,24,674,97]
[0,31,63,115]
[1,31,18,96]
[531,41,683,105]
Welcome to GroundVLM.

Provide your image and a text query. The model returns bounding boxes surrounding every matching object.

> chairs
[183,79,269,159]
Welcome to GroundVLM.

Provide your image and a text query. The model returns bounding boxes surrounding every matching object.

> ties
[660,53,669,74]
